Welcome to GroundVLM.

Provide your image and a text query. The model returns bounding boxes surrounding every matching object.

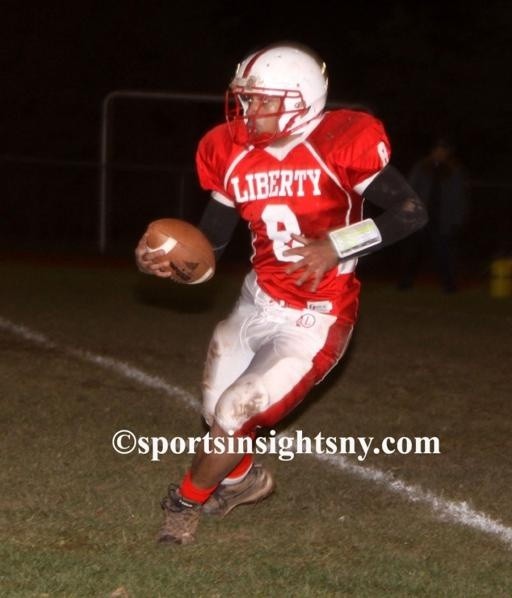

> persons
[393,134,472,295]
[130,40,433,548]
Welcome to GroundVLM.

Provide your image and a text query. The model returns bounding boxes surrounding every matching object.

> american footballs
[147,218,215,284]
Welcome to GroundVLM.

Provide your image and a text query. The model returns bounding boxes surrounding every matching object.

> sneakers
[153,482,207,550]
[201,463,275,523]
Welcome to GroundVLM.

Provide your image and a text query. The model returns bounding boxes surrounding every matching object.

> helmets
[228,44,329,134]
